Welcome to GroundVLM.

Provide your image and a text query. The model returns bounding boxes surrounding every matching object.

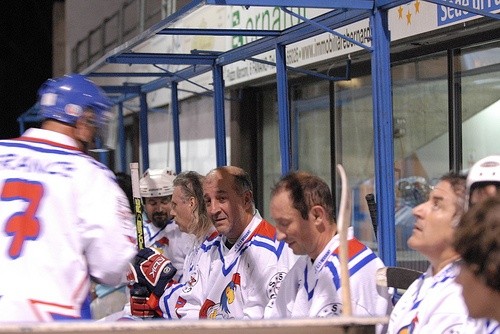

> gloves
[128,283,161,320]
[128,247,178,296]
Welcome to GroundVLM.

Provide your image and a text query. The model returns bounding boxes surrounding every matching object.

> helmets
[140,168,177,204]
[36,73,114,127]
[463,156,500,208]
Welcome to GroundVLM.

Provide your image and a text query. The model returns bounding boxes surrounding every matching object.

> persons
[0,74,138,321]
[98,168,194,321]
[130,171,218,321]
[175,166,301,320]
[271,172,392,334]
[388,155,500,334]
